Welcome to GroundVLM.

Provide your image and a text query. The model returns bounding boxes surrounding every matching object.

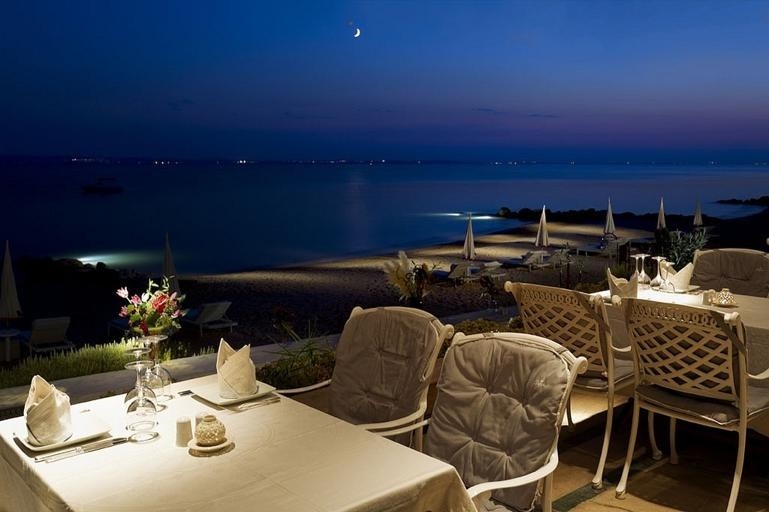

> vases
[135,328,165,363]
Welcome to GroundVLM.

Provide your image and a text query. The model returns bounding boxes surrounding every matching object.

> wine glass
[650,256,667,300]
[135,336,165,407]
[123,360,159,442]
[123,347,157,431]
[635,254,651,291]
[659,262,676,302]
[629,255,641,290]
[143,335,173,402]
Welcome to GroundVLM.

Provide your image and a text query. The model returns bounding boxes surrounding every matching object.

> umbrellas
[533,204,551,251]
[692,199,703,226]
[0,238,22,365]
[160,229,181,297]
[655,195,667,230]
[602,196,616,234]
[460,210,477,261]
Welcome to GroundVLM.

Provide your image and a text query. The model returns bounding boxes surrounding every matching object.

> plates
[13,420,112,451]
[712,301,737,307]
[187,436,231,452]
[651,284,702,292]
[190,379,275,406]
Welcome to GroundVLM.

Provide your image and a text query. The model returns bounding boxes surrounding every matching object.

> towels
[21,372,74,447]
[604,266,638,299]
[659,260,694,291]
[211,336,259,401]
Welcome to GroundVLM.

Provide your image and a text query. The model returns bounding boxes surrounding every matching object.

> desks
[0,328,18,364]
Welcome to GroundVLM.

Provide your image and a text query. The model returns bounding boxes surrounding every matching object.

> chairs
[17,314,78,358]
[686,247,767,299]
[502,280,663,492]
[105,313,129,340]
[370,329,589,512]
[428,237,626,288]
[609,294,768,512]
[176,297,240,337]
[271,304,454,454]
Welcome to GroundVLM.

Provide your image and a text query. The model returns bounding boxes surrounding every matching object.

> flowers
[112,274,184,335]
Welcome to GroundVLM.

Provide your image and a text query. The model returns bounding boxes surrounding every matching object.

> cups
[702,291,712,305]
[176,416,193,447]
[709,288,716,304]
